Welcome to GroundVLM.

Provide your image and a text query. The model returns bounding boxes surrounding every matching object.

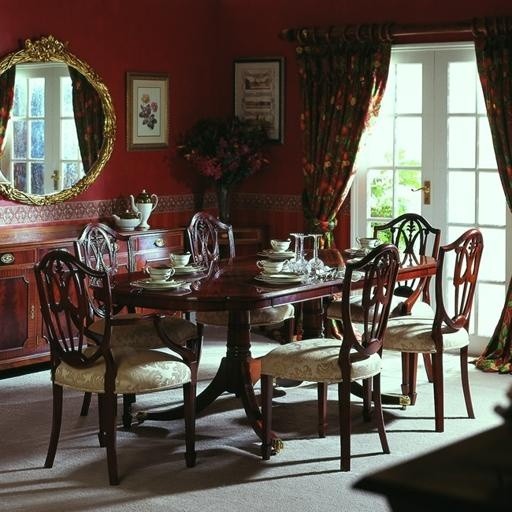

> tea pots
[113,210,143,231]
[128,189,159,228]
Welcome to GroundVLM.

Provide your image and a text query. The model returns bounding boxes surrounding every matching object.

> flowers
[176,115,280,183]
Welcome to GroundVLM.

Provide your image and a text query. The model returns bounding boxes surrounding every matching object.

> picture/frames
[125,71,170,153]
[231,56,287,145]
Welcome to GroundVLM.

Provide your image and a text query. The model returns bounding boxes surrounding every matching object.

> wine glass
[288,232,326,280]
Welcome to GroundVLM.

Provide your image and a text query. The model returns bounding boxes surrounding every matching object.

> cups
[256,259,289,274]
[169,252,191,267]
[146,265,176,282]
[355,237,381,248]
[270,239,291,252]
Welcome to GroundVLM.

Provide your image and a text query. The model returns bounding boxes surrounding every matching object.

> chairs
[322,213,440,384]
[184,213,295,373]
[260,243,400,472]
[362,229,483,432]
[74,222,204,428]
[34,251,198,485]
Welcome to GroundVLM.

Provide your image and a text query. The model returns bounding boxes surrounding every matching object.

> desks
[92,248,438,453]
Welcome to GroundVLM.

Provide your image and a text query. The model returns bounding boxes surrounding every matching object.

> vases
[217,182,233,225]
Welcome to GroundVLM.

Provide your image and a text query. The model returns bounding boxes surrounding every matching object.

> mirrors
[0,34,118,206]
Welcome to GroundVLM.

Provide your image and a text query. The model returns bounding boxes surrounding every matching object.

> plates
[132,278,188,290]
[257,249,308,259]
[344,248,394,268]
[255,272,302,285]
[172,265,207,275]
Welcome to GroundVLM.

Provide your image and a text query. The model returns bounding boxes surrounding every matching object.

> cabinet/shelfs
[0,224,269,371]
